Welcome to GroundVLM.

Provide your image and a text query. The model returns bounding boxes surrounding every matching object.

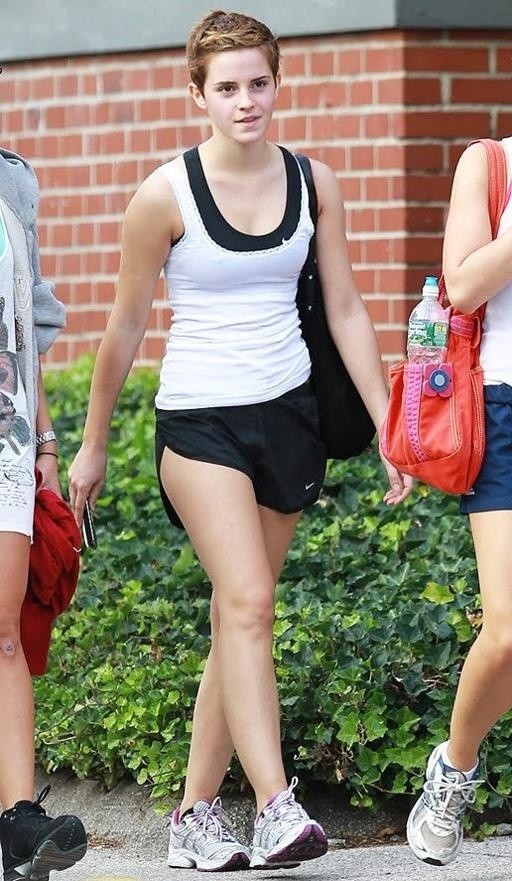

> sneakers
[0,800,90,881]
[406,739,479,866]
[167,789,328,872]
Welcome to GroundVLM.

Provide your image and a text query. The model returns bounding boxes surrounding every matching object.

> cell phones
[80,499,98,550]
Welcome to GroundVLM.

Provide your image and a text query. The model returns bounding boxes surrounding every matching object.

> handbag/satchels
[295,157,377,461]
[379,138,508,496]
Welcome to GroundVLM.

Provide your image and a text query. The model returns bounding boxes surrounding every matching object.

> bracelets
[36,431,57,447]
[38,451,58,458]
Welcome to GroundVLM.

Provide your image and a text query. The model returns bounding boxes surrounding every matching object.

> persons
[402,129,512,867]
[0,143,94,881]
[61,8,415,873]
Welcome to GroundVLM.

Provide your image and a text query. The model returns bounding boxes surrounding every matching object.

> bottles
[406,273,446,370]
